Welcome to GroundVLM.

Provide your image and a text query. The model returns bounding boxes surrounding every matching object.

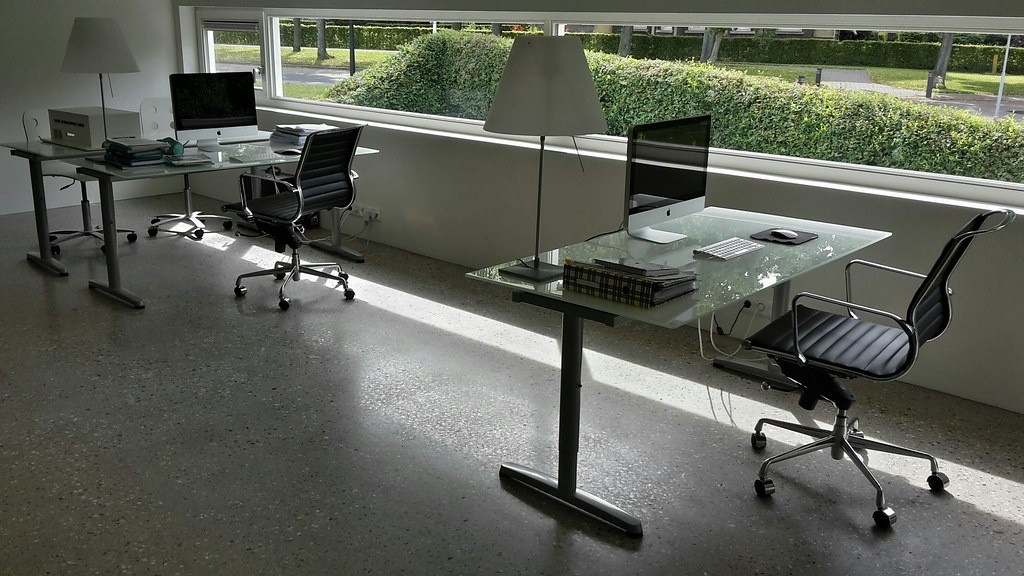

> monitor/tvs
[623,114,711,242]
[168,71,259,153]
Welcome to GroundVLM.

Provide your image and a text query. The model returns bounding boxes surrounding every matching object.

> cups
[162,138,184,154]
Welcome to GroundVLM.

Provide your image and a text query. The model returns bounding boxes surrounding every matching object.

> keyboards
[693,236,765,261]
[228,152,285,163]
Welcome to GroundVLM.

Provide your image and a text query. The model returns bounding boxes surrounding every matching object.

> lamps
[482,35,609,283]
[139,97,235,238]
[60,16,140,164]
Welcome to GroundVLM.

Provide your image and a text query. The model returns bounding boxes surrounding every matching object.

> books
[593,257,680,277]
[85,138,215,170]
[269,123,340,150]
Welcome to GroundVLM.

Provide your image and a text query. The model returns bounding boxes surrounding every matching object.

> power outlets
[339,204,361,217]
[359,206,383,222]
[738,296,771,318]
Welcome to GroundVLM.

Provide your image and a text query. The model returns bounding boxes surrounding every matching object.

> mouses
[282,148,301,155]
[771,229,798,239]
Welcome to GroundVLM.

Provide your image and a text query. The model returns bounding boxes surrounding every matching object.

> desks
[0,130,270,277]
[464,205,893,538]
[59,132,381,310]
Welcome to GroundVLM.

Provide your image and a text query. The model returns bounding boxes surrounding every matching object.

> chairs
[233,125,369,311]
[22,108,137,256]
[742,207,1016,528]
